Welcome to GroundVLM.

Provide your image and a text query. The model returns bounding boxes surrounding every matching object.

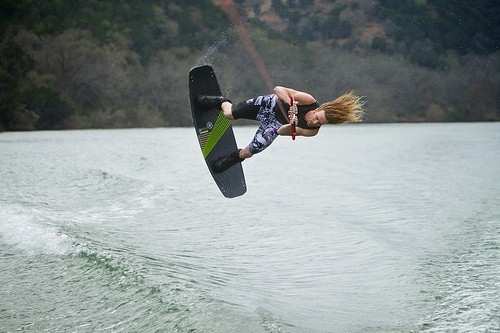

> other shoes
[214,150,239,172]
[197,95,224,110]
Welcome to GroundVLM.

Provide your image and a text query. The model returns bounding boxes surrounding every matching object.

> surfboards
[187,65,247,199]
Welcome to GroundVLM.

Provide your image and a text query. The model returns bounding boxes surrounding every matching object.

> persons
[197,86,367,173]
